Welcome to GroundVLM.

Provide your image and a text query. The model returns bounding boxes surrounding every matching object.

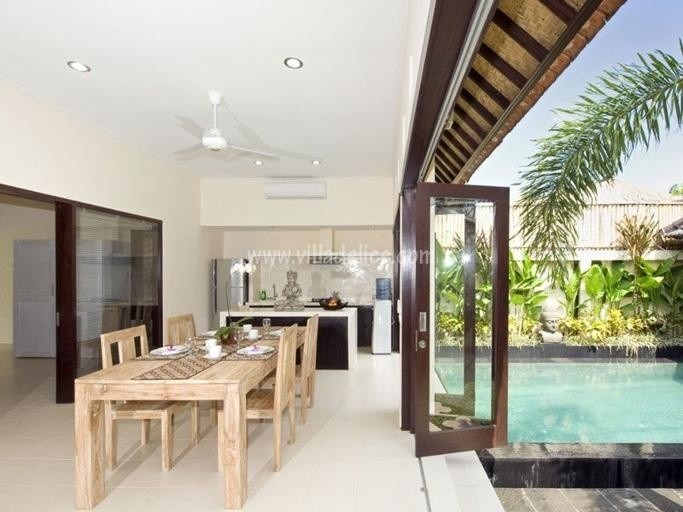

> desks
[217,306,358,371]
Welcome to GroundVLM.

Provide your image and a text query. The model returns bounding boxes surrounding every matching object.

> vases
[219,327,239,345]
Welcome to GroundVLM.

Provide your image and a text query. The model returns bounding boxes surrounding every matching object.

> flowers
[213,258,256,341]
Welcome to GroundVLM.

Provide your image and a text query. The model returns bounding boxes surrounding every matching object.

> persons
[275,270,304,311]
[537,298,565,342]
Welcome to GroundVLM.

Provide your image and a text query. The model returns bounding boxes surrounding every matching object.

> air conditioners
[263,179,325,199]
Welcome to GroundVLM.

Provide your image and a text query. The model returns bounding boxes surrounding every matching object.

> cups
[205,338,217,352]
[243,324,251,332]
[207,345,222,357]
[248,330,258,338]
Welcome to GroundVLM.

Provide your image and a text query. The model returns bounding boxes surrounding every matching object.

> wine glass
[233,327,244,352]
[261,318,270,339]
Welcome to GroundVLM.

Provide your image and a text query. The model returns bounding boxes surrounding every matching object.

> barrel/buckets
[376,278,392,300]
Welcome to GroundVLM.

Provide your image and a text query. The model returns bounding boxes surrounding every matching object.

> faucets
[272,284,277,299]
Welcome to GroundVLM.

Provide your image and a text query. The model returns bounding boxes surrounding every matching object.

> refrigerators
[209,258,248,330]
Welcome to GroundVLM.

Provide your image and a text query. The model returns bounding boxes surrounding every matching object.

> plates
[150,344,189,356]
[196,345,222,349]
[244,335,261,339]
[197,330,219,337]
[203,353,227,359]
[235,345,274,355]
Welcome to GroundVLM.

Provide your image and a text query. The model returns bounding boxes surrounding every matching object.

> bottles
[260,287,265,300]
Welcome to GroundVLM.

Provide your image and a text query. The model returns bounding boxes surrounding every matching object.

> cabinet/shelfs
[355,305,373,347]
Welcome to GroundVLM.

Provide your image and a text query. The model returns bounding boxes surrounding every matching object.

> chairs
[99,323,200,473]
[255,313,320,428]
[215,322,298,473]
[160,313,216,428]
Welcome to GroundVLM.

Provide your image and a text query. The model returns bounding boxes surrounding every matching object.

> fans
[169,88,278,160]
